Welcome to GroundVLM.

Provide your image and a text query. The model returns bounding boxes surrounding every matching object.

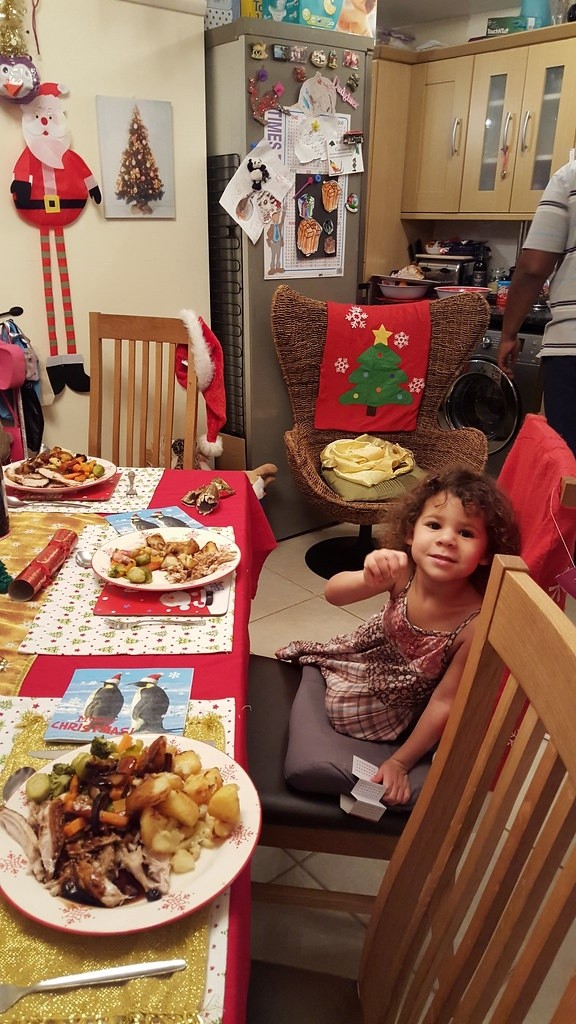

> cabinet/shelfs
[358,19,576,302]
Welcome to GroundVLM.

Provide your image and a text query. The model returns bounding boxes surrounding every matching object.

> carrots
[109,560,161,578]
[62,735,133,834]
[64,457,97,482]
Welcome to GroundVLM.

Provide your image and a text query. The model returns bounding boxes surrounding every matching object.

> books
[42,668,195,746]
[105,506,210,536]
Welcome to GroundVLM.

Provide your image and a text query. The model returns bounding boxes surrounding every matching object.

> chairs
[246,414,576,861]
[89,309,227,471]
[270,284,490,580]
[244,554,576,1024]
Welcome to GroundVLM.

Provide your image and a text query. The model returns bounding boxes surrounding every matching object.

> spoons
[75,550,95,568]
[0,767,36,810]
[6,496,91,509]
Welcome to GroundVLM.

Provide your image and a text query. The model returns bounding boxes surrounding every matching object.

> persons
[274,462,521,804]
[497,159,576,459]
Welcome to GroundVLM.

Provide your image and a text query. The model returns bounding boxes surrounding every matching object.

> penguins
[126,681,171,735]
[73,680,124,731]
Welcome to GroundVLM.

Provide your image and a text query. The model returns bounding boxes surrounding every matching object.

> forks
[125,471,137,498]
[103,617,205,630]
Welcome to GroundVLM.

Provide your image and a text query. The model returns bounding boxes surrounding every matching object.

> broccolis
[48,735,141,798]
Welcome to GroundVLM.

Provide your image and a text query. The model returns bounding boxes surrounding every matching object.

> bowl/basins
[434,286,493,300]
[377,283,429,299]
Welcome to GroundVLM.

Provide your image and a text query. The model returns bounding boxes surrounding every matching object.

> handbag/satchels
[0,322,25,389]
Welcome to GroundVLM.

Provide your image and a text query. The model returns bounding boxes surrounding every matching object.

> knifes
[28,750,74,760]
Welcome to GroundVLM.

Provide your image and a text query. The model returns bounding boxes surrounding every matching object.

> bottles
[471,250,487,287]
[487,267,508,306]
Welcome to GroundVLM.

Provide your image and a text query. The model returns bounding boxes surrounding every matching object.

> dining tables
[0,446,277,1024]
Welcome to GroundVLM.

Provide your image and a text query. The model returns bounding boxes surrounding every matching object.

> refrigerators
[204,16,374,539]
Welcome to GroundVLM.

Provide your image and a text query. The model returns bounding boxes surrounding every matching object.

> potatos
[125,748,239,872]
[144,533,218,568]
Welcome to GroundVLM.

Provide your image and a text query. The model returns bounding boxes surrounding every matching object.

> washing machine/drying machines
[437,331,543,481]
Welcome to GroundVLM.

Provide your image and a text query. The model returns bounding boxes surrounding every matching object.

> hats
[174,309,226,457]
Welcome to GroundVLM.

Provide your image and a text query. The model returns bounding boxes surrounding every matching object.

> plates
[0,732,262,937]
[1,455,117,494]
[91,527,241,592]
